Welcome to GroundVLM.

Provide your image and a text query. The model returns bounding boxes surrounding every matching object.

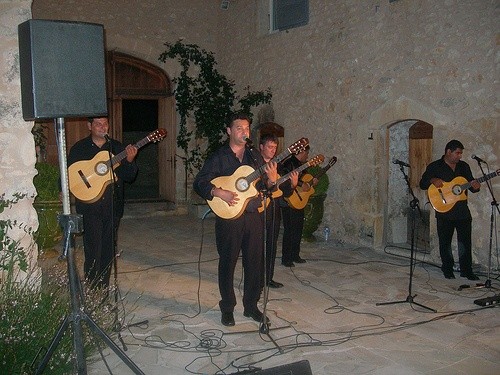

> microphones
[471,154,487,164]
[102,133,111,143]
[243,136,254,147]
[393,159,411,167]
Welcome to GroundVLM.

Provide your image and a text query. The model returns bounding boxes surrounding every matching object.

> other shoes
[220,311,236,327]
[290,256,307,263]
[281,261,295,268]
[243,308,270,323]
[444,271,455,279]
[267,280,284,288]
[460,271,480,280]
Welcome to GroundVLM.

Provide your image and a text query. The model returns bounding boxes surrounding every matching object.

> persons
[67,116,137,303]
[280,146,319,267]
[419,139,481,281]
[256,133,299,288]
[193,114,277,328]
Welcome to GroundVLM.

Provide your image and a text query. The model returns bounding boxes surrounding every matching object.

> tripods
[223,145,291,353]
[35,118,148,375]
[376,165,437,314]
[474,160,500,292]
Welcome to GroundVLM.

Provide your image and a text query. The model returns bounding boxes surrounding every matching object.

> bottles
[324,226,329,240]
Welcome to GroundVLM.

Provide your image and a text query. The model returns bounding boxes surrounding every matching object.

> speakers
[242,360,312,375]
[17,18,109,122]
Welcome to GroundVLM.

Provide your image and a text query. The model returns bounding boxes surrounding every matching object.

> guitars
[428,169,500,213]
[207,137,310,219]
[68,128,168,204]
[258,153,326,214]
[284,155,338,210]
